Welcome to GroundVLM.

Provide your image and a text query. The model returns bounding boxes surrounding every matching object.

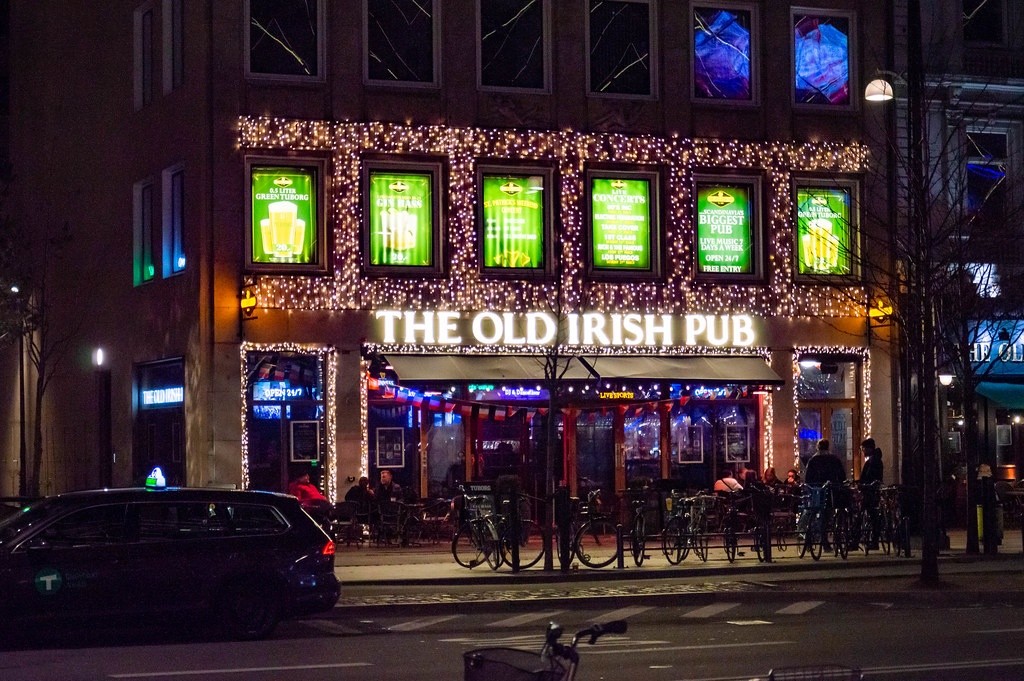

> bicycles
[452,479,546,571]
[463,620,627,681]
[618,478,902,566]
[556,477,618,567]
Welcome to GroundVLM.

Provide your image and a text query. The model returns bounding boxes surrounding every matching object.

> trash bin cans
[976,504,1004,545]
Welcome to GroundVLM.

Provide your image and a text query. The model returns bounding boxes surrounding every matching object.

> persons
[977,464,1003,545]
[289,468,330,506]
[375,470,403,495]
[714,468,804,492]
[847,438,883,551]
[344,477,374,524]
[805,438,847,552]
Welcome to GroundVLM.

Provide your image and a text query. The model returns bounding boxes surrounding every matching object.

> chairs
[310,485,455,548]
[706,484,813,541]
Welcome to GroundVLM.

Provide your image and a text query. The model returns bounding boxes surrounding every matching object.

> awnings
[382,355,785,386]
[973,379,1024,411]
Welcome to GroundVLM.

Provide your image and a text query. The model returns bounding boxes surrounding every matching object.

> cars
[0,487,342,638]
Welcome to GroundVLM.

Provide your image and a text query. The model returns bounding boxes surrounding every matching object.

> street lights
[11,282,27,497]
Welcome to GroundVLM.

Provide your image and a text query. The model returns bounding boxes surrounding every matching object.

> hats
[861,438,875,450]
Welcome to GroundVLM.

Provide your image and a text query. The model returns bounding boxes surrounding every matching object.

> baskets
[801,486,831,510]
